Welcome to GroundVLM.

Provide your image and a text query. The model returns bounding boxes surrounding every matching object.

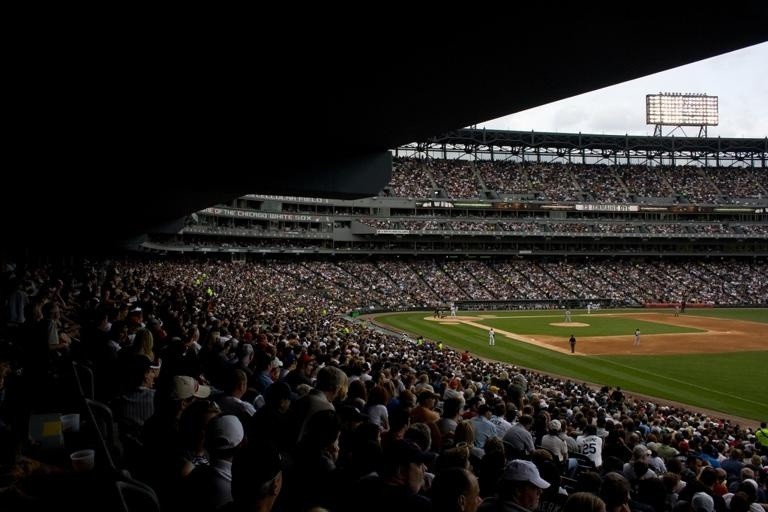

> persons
[569,334,576,353]
[634,329,640,346]
[0,155,768,512]
[488,328,496,347]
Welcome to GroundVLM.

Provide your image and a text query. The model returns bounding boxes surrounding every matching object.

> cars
[61,413,81,435]
[70,449,95,476]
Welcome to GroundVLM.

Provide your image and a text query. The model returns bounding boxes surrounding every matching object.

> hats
[125,301,768,512]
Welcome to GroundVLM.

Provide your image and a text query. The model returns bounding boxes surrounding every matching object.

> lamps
[86,397,161,510]
